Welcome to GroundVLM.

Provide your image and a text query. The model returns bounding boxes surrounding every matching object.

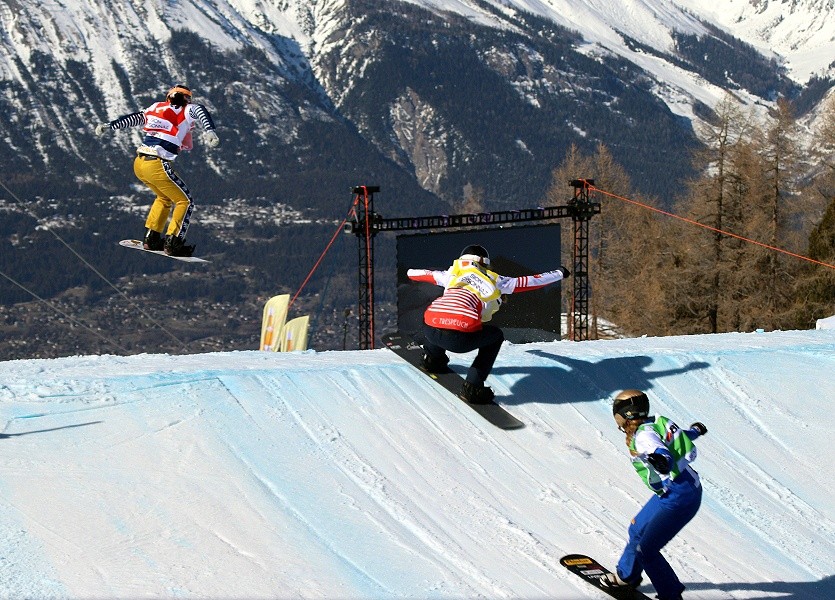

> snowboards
[379,331,526,432]
[118,239,214,264]
[559,553,653,600]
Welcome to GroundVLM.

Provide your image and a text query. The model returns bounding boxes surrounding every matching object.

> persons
[394,244,571,403]
[94,84,220,256]
[600,389,707,600]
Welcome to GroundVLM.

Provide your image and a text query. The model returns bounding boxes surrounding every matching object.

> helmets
[613,389,649,427]
[460,244,490,267]
[166,84,193,104]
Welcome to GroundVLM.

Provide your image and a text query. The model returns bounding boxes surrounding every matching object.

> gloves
[205,131,220,148]
[95,123,110,136]
[556,266,570,278]
[690,422,707,435]
[647,453,670,474]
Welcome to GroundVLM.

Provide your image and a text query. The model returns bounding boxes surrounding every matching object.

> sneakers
[164,236,194,256]
[460,380,495,403]
[144,232,166,251]
[419,352,450,373]
[599,572,643,589]
